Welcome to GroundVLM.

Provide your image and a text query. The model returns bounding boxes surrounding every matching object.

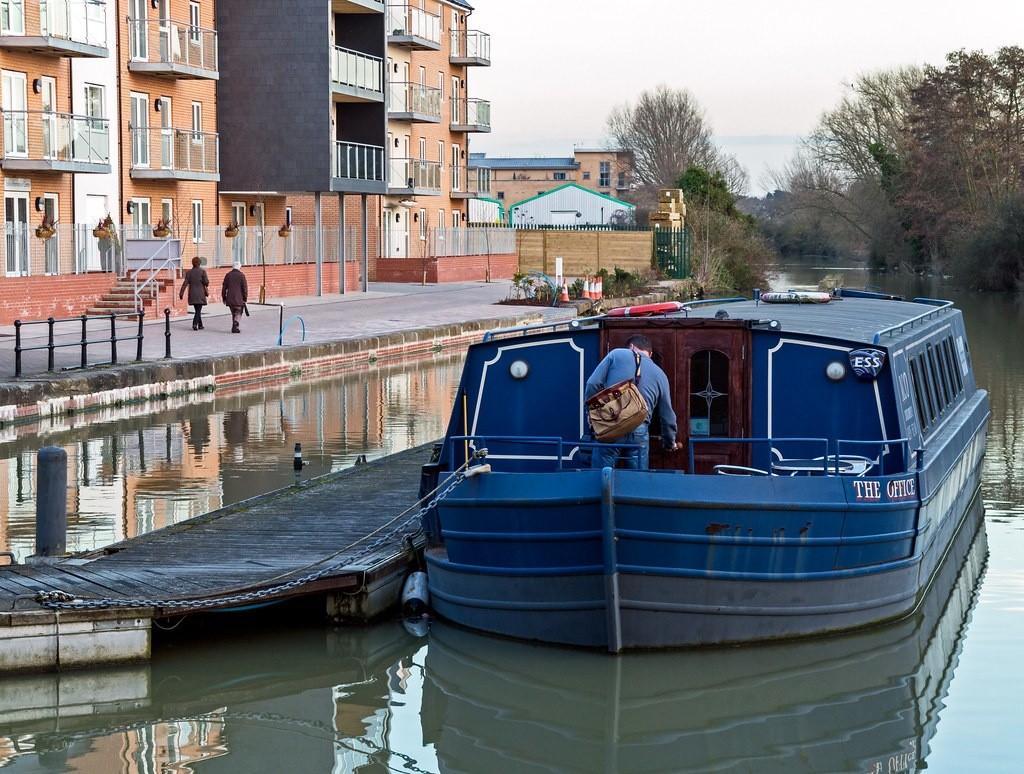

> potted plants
[152,219,169,237]
[36,215,57,237]
[279,223,290,236]
[93,212,115,237]
[225,223,240,237]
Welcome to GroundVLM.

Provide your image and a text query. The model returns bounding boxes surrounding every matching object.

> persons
[179,257,209,330]
[585,336,678,471]
[221,261,248,333]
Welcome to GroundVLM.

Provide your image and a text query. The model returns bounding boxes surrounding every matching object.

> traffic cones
[559,277,573,302]
[580,276,603,300]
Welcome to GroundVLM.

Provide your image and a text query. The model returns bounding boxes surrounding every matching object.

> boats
[419,286,992,656]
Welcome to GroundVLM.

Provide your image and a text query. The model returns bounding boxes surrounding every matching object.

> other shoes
[232,329,240,333]
[198,327,204,329]
[193,325,197,330]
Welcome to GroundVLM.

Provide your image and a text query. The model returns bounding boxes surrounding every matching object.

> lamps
[396,212,400,221]
[461,14,464,22]
[461,149,465,158]
[155,98,162,110]
[34,196,42,211]
[126,200,133,213]
[394,63,398,72]
[462,212,466,220]
[414,212,418,222]
[249,205,255,215]
[461,79,464,87]
[150,0,158,8]
[394,138,398,147]
[32,79,41,93]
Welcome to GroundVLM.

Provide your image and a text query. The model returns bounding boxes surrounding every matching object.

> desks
[771,459,854,477]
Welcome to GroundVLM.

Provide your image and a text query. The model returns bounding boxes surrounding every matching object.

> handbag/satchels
[585,380,648,444]
[205,288,209,297]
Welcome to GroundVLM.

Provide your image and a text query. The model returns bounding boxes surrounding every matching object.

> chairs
[790,454,874,478]
[712,464,780,477]
